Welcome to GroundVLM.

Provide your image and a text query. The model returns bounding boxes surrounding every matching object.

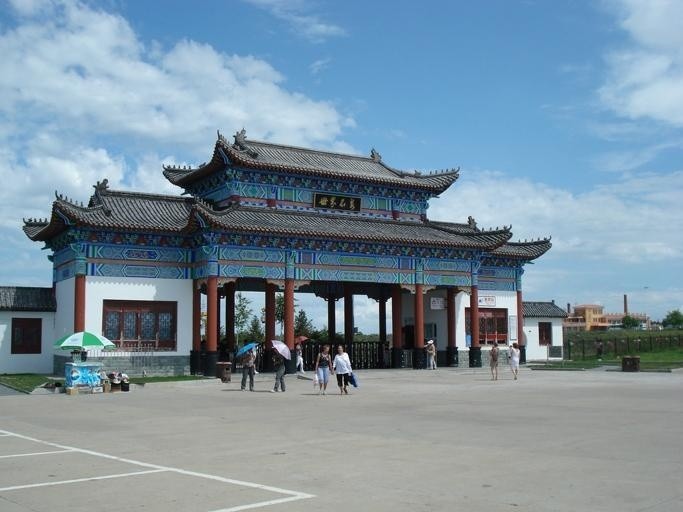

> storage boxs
[65,374,129,396]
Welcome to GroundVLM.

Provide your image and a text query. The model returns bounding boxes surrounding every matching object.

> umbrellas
[270,339,291,361]
[51,331,117,362]
[294,335,308,344]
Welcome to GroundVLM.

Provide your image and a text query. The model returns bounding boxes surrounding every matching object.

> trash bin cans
[216,361,232,383]
[622,356,640,372]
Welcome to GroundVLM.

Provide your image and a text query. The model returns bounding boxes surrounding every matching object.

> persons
[331,344,352,395]
[315,344,333,395]
[270,347,286,393]
[200,336,264,375]
[295,343,305,373]
[489,343,498,381]
[240,347,256,393]
[509,343,520,380]
[425,340,437,370]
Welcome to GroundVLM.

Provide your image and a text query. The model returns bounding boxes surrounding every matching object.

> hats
[427,340,433,344]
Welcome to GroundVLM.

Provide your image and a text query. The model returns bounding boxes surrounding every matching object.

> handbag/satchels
[312,374,318,386]
[350,373,357,387]
